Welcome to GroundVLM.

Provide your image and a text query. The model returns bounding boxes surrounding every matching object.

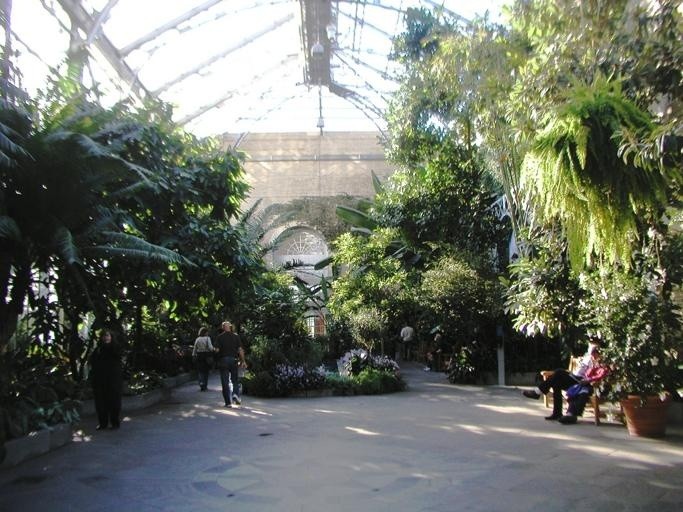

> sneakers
[559,415,577,423]
[545,413,562,420]
[233,394,241,404]
[523,391,540,400]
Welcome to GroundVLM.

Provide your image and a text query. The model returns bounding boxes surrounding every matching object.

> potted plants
[597,286,679,441]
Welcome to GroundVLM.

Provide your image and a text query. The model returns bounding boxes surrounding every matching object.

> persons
[399,321,415,360]
[521,336,605,422]
[86,329,130,431]
[557,346,609,425]
[191,326,216,392]
[423,333,444,372]
[213,320,248,407]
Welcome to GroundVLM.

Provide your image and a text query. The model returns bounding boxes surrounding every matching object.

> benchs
[539,355,628,426]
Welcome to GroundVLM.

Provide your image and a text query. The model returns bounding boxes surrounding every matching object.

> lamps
[315,81,324,136]
[309,6,325,61]
